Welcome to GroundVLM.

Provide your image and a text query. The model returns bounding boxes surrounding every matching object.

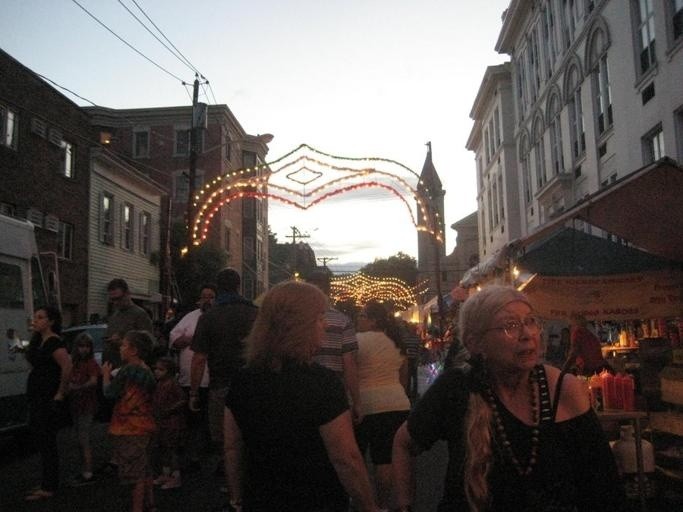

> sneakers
[161,470,182,489]
[153,466,170,485]
[70,471,95,487]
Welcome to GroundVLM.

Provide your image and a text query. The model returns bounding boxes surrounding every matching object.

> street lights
[184,131,274,249]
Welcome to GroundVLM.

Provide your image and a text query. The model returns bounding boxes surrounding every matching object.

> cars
[58,323,109,368]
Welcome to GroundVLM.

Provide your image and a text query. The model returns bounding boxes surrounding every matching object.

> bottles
[588,367,637,413]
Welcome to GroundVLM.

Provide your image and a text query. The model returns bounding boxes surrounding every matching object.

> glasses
[487,313,542,339]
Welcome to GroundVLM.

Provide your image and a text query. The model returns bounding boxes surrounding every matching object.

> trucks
[0,208,68,453]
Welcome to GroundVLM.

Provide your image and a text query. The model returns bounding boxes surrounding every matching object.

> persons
[562,314,602,378]
[394,285,627,512]
[26,265,423,512]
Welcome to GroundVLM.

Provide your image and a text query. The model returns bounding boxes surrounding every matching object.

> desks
[601,347,640,357]
[595,411,648,512]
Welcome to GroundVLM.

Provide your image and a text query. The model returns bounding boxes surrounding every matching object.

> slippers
[24,485,54,502]
